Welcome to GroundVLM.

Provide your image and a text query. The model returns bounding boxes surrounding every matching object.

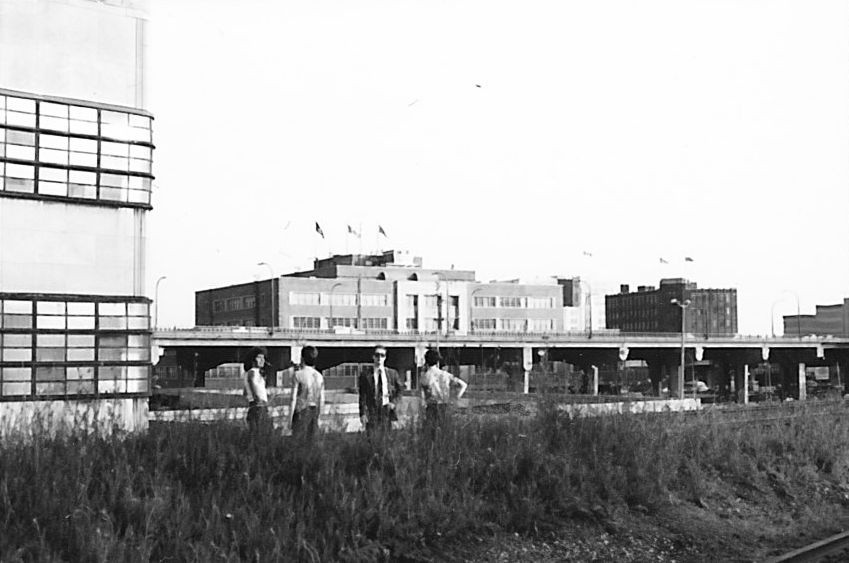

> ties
[378,369,383,408]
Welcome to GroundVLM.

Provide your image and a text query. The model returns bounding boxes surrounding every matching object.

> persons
[287,345,325,434]
[358,345,404,433]
[243,350,268,429]
[419,350,468,427]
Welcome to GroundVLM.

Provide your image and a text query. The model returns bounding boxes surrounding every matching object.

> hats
[425,350,443,359]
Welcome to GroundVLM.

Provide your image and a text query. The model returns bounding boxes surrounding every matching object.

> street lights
[257,262,276,332]
[328,283,342,333]
[153,276,167,332]
[431,272,449,338]
[670,298,691,400]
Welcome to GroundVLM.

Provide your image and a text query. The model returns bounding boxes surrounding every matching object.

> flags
[660,258,668,263]
[379,226,386,236]
[686,256,694,262]
[316,222,323,238]
[348,226,358,234]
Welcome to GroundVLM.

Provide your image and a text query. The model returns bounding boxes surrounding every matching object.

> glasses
[374,352,386,358]
